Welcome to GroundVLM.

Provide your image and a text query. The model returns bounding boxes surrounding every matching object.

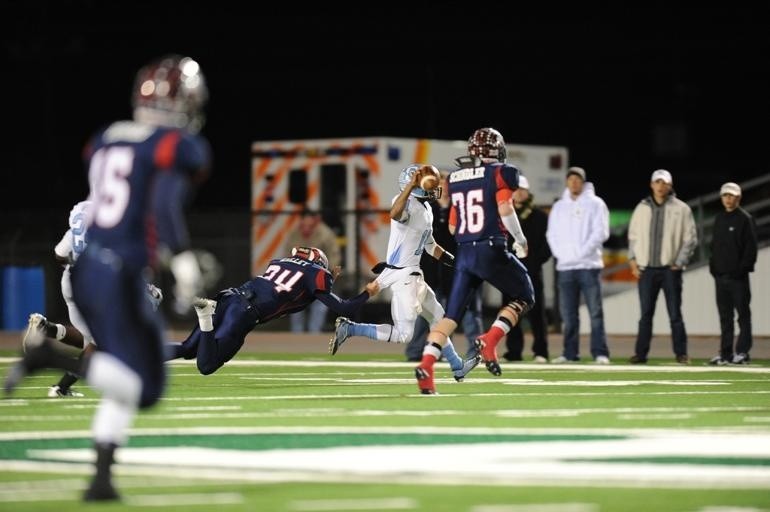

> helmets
[130,54,209,128]
[397,164,443,202]
[467,126,508,165]
[289,244,329,268]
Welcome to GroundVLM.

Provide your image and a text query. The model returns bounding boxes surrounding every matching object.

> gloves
[144,283,163,308]
[169,245,225,320]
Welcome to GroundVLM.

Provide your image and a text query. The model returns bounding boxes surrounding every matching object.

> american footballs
[417,165,441,190]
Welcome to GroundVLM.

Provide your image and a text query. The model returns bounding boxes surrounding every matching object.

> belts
[232,287,261,326]
[456,236,506,248]
[410,271,421,276]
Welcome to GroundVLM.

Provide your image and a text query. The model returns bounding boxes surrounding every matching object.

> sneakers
[595,354,611,366]
[82,471,124,502]
[416,355,438,395]
[5,335,61,393]
[23,313,49,355]
[628,356,648,363]
[676,354,693,365]
[328,316,349,357]
[551,355,581,364]
[532,354,546,365]
[47,384,86,399]
[704,352,749,365]
[193,295,217,318]
[475,326,506,376]
[451,356,481,383]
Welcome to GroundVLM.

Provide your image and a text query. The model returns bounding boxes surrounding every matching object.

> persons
[163,246,380,376]
[499,174,552,366]
[708,183,758,365]
[544,167,611,365]
[23,192,164,396]
[3,50,213,501]
[328,164,482,383]
[404,171,484,362]
[628,169,698,366]
[415,127,536,395]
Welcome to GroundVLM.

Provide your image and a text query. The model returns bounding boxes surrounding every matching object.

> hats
[719,182,742,198]
[567,166,586,181]
[519,174,531,190]
[650,169,672,186]
